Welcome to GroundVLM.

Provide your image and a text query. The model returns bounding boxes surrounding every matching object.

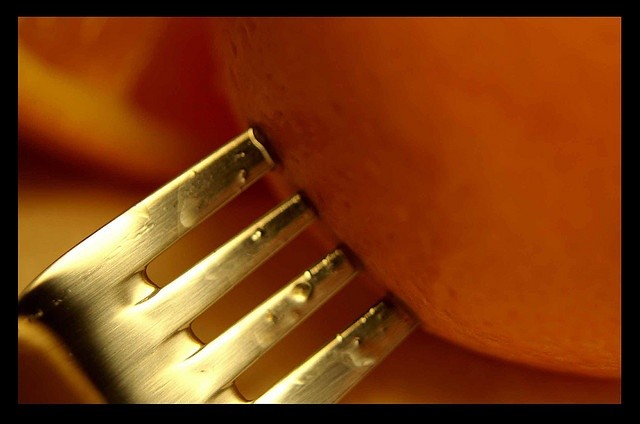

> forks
[17,128,422,407]
